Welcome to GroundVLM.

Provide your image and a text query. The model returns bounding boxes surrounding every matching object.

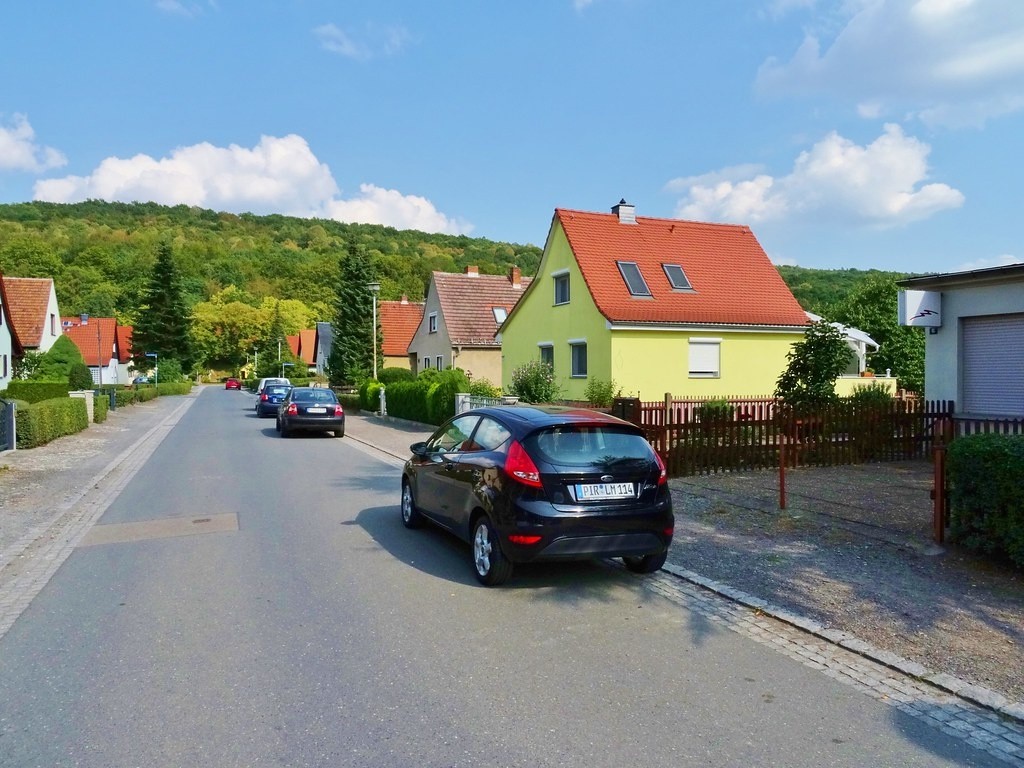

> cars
[226,378,241,391]
[401,405,675,587]
[276,386,345,438]
[255,384,294,418]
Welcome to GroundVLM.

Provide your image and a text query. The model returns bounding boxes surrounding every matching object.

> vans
[257,378,290,399]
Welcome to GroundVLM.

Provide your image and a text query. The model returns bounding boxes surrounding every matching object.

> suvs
[133,376,150,384]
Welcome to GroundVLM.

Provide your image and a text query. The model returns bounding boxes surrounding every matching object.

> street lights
[253,347,258,368]
[367,281,381,380]
